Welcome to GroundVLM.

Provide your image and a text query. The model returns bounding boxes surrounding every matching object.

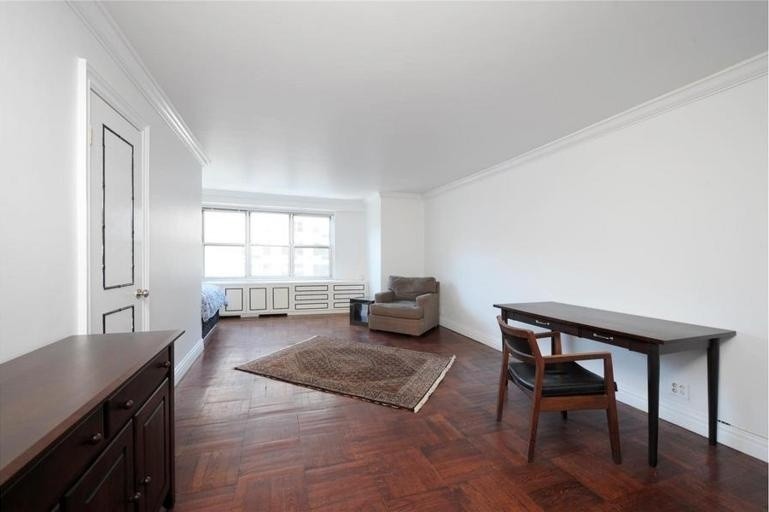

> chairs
[494,315,624,464]
[368,275,441,336]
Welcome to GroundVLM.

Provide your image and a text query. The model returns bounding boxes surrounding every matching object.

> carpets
[234,335,457,414]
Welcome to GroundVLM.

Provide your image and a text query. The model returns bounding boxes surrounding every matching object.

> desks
[492,302,737,466]
[350,296,375,327]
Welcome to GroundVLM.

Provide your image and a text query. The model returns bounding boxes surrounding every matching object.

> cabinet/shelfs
[0,329,186,512]
